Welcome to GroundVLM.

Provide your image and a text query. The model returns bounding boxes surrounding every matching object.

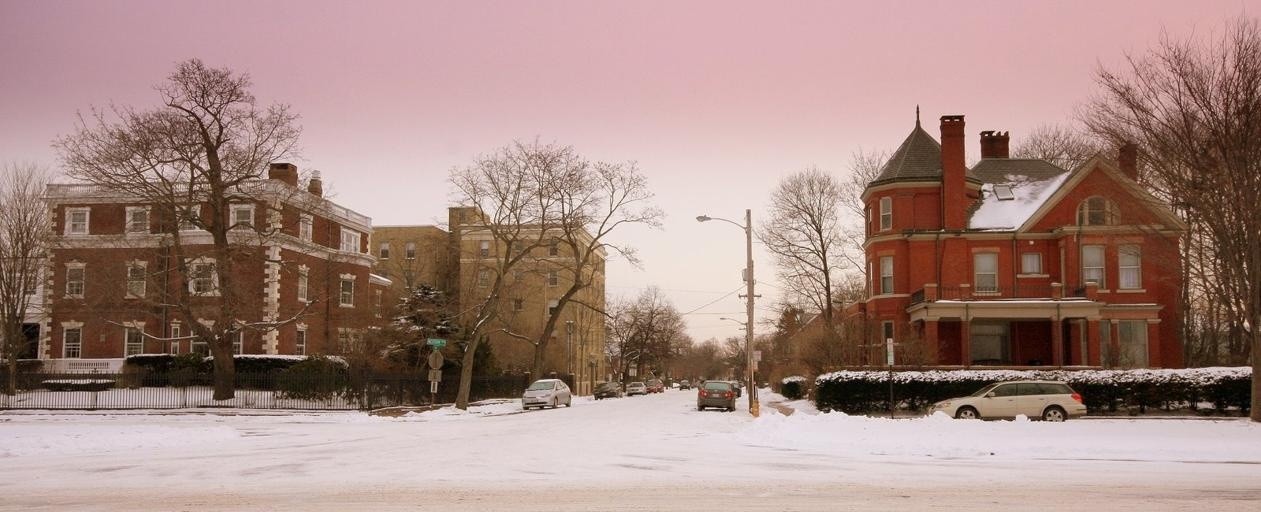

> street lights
[695,209,754,413]
[720,317,747,325]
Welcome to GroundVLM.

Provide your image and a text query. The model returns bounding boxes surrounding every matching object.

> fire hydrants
[752,397,760,417]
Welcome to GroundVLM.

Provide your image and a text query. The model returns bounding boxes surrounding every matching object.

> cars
[593,379,664,400]
[666,377,701,390]
[928,380,1087,422]
[697,379,745,411]
[522,379,571,409]
